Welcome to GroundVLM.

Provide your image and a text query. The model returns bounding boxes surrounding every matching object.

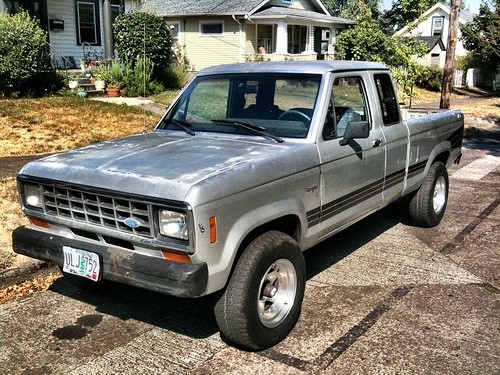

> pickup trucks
[11,58,465,353]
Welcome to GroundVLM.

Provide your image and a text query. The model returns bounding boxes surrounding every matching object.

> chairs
[284,108,314,127]
[239,104,279,122]
[329,107,354,139]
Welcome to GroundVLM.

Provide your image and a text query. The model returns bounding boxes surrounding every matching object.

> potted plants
[106,86,120,95]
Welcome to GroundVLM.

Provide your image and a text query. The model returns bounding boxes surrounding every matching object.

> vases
[95,81,105,91]
[70,82,78,89]
